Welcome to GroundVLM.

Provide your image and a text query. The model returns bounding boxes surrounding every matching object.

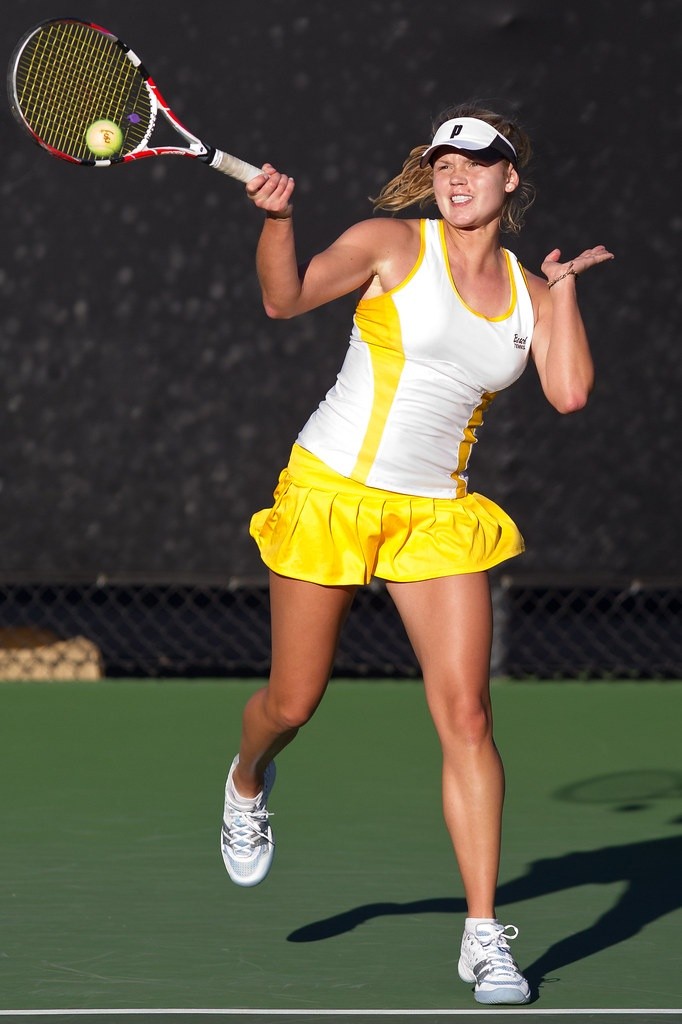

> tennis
[86,119,121,156]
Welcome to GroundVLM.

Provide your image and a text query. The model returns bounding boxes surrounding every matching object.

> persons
[220,105,612,1003]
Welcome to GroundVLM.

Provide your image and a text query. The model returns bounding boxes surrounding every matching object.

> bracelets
[546,262,580,290]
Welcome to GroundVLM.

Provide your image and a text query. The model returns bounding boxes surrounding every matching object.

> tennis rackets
[10,19,262,185]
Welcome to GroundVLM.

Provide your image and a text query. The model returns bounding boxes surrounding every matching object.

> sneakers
[457,923,531,1004]
[220,753,276,887]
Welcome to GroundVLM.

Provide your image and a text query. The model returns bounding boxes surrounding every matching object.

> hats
[419,117,518,172]
